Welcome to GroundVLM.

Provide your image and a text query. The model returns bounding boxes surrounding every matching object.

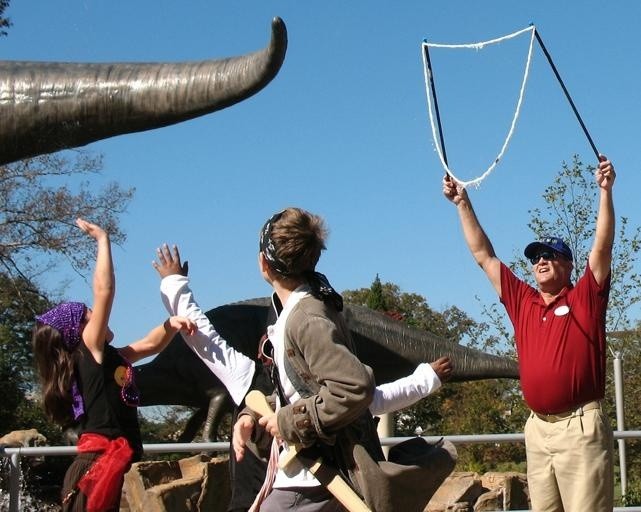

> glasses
[531,250,559,264]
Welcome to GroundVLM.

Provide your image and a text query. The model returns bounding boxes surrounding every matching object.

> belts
[534,400,600,422]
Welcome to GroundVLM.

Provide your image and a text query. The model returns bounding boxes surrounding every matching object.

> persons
[232,208,392,512]
[443,153,615,512]
[152,243,454,512]
[32,218,198,512]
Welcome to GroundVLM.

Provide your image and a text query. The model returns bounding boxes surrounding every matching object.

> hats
[524,237,572,261]
